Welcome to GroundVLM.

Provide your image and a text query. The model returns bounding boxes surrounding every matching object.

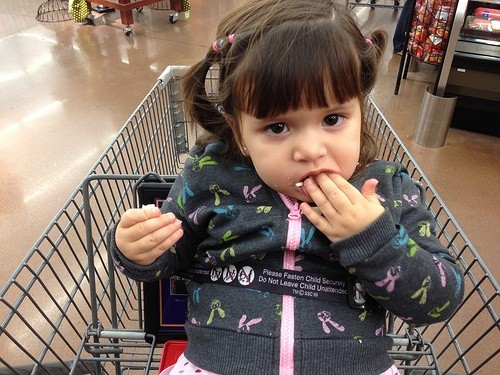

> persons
[106,0,465,375]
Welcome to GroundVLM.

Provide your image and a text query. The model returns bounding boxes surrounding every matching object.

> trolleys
[84,0,183,36]
[345,0,407,11]
[0,64,500,375]
